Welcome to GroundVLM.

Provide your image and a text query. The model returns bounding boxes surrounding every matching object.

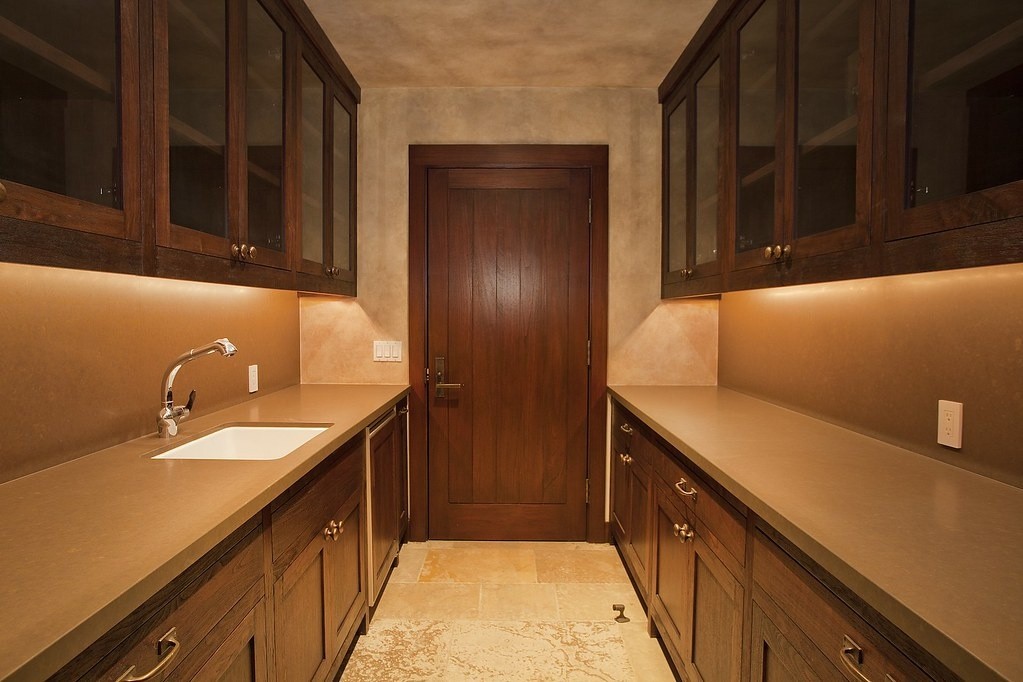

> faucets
[155,337,239,439]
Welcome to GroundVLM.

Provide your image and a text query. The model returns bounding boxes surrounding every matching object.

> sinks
[139,421,335,461]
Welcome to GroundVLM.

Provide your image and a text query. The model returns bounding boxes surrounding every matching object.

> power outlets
[938,399,964,449]
[248,365,259,393]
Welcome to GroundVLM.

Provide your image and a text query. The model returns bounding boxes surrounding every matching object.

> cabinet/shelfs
[658,1,1023,300]
[43,395,408,682]
[610,395,933,682]
[0,0,360,297]
